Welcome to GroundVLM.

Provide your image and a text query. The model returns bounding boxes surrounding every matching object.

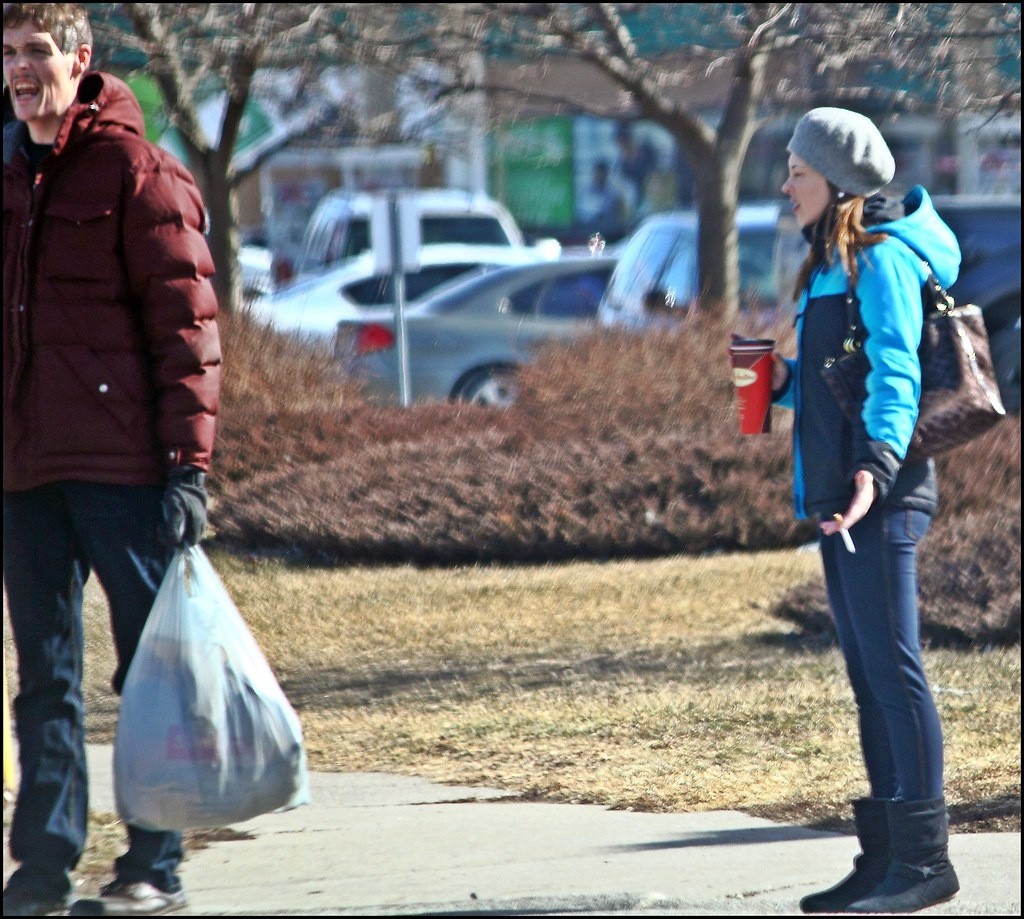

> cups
[729,337,773,435]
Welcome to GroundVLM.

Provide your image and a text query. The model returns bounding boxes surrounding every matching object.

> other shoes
[1,860,77,915]
[70,879,189,918]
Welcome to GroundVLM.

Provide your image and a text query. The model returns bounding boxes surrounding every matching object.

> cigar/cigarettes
[833,512,856,554]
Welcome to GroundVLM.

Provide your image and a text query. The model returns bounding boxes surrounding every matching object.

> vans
[599,203,783,327]
[294,184,521,285]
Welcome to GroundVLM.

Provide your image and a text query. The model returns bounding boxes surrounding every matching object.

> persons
[3,2,222,916]
[725,107,961,913]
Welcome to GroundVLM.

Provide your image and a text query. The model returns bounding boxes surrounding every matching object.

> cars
[244,244,532,350]
[901,197,1022,391]
[334,255,625,415]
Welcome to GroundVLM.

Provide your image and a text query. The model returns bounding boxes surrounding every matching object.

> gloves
[152,465,209,549]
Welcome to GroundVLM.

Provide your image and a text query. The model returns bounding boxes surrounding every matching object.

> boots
[799,797,890,911]
[846,798,959,913]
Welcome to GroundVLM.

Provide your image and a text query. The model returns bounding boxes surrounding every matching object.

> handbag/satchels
[820,227,1007,468]
[114,544,309,832]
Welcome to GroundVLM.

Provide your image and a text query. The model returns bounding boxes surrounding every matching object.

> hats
[787,107,896,198]
